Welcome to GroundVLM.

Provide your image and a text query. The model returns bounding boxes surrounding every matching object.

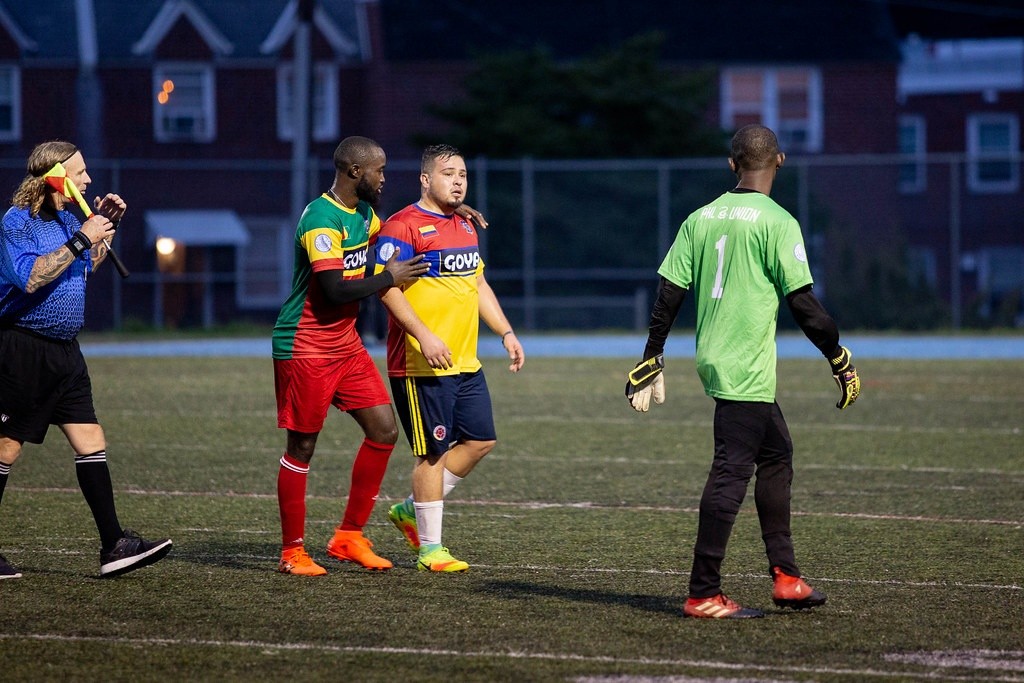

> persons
[270,136,489,576]
[626,124,862,619]
[0,142,171,578]
[371,144,525,573]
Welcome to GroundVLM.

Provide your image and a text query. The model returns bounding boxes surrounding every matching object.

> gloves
[828,347,861,409]
[625,353,665,412]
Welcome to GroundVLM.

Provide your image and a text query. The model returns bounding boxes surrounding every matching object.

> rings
[478,213,482,217]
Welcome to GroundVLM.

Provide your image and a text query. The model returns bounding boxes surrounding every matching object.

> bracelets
[64,231,93,258]
[501,331,511,348]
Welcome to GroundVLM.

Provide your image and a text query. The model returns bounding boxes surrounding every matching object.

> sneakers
[385,498,420,554]
[683,592,765,618]
[100,529,173,578]
[278,547,328,577]
[326,527,393,571]
[0,553,22,579]
[771,567,827,610]
[416,544,469,572]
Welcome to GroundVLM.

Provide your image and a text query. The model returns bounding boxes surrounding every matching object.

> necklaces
[329,188,348,208]
